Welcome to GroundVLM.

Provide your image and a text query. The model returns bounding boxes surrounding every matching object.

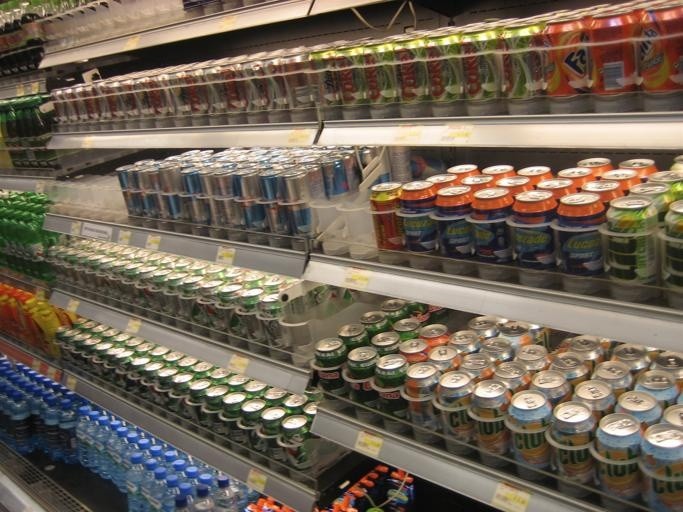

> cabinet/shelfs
[45,0,362,512]
[0,0,46,512]
[309,0,683,512]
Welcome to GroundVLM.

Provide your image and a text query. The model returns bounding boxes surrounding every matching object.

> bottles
[112,427,129,484]
[149,446,164,466]
[127,454,145,512]
[499,323,531,352]
[470,188,514,265]
[185,68,209,114]
[157,367,180,419]
[112,259,133,309]
[491,361,531,395]
[146,167,171,218]
[638,423,683,511]
[87,411,101,471]
[163,351,185,369]
[197,474,215,500]
[117,244,187,272]
[159,474,181,512]
[74,333,91,368]
[380,299,409,325]
[284,394,310,416]
[662,289,683,309]
[401,180,437,254]
[12,392,34,454]
[62,329,82,363]
[95,341,114,384]
[263,55,290,110]
[136,439,152,459]
[634,370,681,408]
[235,169,270,231]
[542,18,590,98]
[425,32,463,103]
[220,284,246,348]
[421,323,452,349]
[561,275,605,295]
[140,459,160,512]
[407,300,431,327]
[112,333,131,348]
[134,147,356,177]
[511,190,559,270]
[164,451,178,475]
[281,170,317,240]
[88,254,107,299]
[259,290,293,362]
[504,20,541,100]
[193,485,215,512]
[47,246,64,286]
[59,399,77,463]
[481,165,516,182]
[185,466,199,486]
[95,416,112,478]
[181,275,204,332]
[547,352,589,392]
[51,88,69,120]
[56,308,77,326]
[517,270,557,289]
[205,384,231,447]
[101,329,121,342]
[149,345,172,362]
[118,165,135,213]
[496,175,533,200]
[129,165,158,215]
[660,404,682,429]
[399,340,430,364]
[108,421,123,478]
[619,158,659,184]
[0,383,11,436]
[429,306,450,322]
[146,73,169,116]
[472,379,509,468]
[609,343,651,383]
[442,258,476,277]
[173,495,191,512]
[126,337,144,351]
[106,80,122,118]
[212,368,232,384]
[529,370,572,407]
[227,374,252,392]
[614,391,662,432]
[185,257,298,291]
[125,261,145,314]
[180,483,194,512]
[241,400,268,463]
[173,460,185,482]
[1,189,53,217]
[446,164,479,182]
[530,326,547,346]
[75,407,90,467]
[177,356,199,373]
[203,65,223,114]
[552,402,595,496]
[338,153,360,193]
[240,57,269,112]
[558,168,596,192]
[427,174,460,191]
[406,362,438,446]
[408,253,440,272]
[536,178,578,204]
[348,346,379,422]
[303,402,319,428]
[131,355,154,406]
[581,179,625,210]
[167,71,190,114]
[663,200,683,286]
[58,248,75,289]
[459,353,495,384]
[265,387,287,408]
[33,387,44,449]
[72,317,90,329]
[609,281,663,303]
[371,332,401,357]
[394,37,428,104]
[348,241,381,263]
[1,355,32,392]
[334,45,368,108]
[393,319,422,341]
[106,346,126,391]
[62,87,78,120]
[219,62,245,113]
[44,2,198,42]
[96,407,261,512]
[165,272,189,325]
[150,467,168,512]
[72,85,86,120]
[360,311,391,339]
[388,147,412,182]
[132,76,151,117]
[591,7,640,96]
[222,393,249,453]
[1,95,54,168]
[181,168,212,222]
[409,156,448,180]
[577,157,613,180]
[199,170,228,227]
[144,362,166,411]
[0,283,56,355]
[93,81,112,119]
[671,155,683,177]
[370,181,404,251]
[337,324,370,352]
[645,170,683,201]
[0,217,48,280]
[601,169,641,195]
[606,195,662,287]
[438,371,473,456]
[311,48,342,108]
[190,380,216,440]
[99,256,118,306]
[509,391,553,481]
[240,288,267,354]
[469,316,500,342]
[173,373,199,430]
[294,280,359,322]
[82,84,98,119]
[139,264,160,320]
[427,346,462,375]
[650,351,682,396]
[378,251,407,268]
[321,236,350,257]
[136,343,156,357]
[66,249,84,293]
[160,162,186,219]
[596,413,642,510]
[628,182,676,231]
[45,397,59,460]
[447,330,481,357]
[30,370,90,405]
[77,251,95,297]
[260,170,293,235]
[365,41,397,106]
[192,361,216,379]
[199,279,226,340]
[55,326,70,360]
[214,477,237,512]
[572,380,616,425]
[116,350,136,398]
[567,336,603,378]
[84,337,102,377]
[513,344,551,379]
[302,164,327,199]
[321,154,349,196]
[304,386,322,401]
[461,24,502,102]
[281,52,314,109]
[48,232,62,245]
[639,1,683,92]
[479,338,512,367]
[316,337,347,414]
[90,324,110,337]
[213,168,245,228]
[591,360,634,401]
[460,174,495,193]
[435,185,476,260]
[262,407,288,462]
[0,0,48,75]
[557,193,606,277]
[63,235,117,258]
[120,432,139,492]
[282,415,315,470]
[5,387,16,442]
[119,78,139,117]
[376,355,406,434]
[516,166,554,189]
[154,269,173,324]
[358,147,391,190]
[478,263,517,281]
[81,321,99,333]
[245,380,269,401]
